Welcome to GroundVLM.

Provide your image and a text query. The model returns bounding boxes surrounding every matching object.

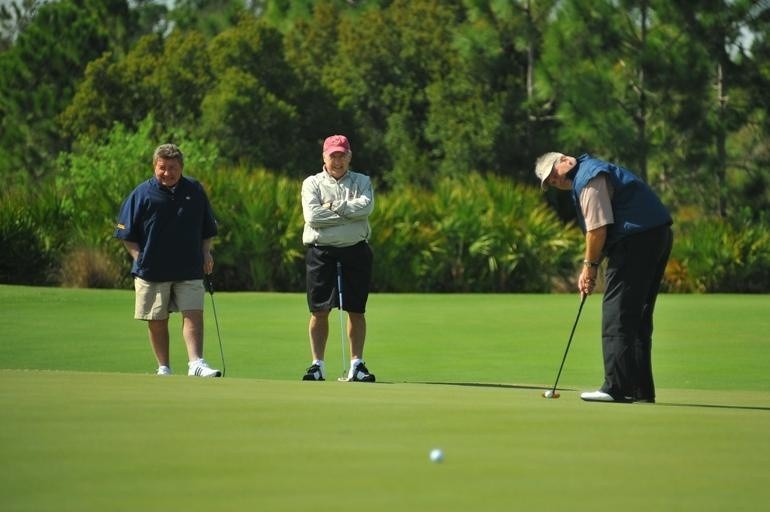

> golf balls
[545,390,553,397]
[430,450,443,462]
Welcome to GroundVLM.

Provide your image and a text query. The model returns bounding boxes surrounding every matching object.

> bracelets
[329,202,332,210]
[581,258,598,267]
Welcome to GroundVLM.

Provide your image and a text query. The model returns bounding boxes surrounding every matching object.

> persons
[115,143,221,376]
[301,134,379,382]
[535,153,674,402]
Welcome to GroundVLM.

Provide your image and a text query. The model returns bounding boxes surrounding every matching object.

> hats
[323,134,349,156]
[541,159,556,192]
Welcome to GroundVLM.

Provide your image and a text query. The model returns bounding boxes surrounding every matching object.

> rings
[208,261,211,264]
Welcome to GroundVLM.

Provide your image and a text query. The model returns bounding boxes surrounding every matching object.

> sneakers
[348,361,375,382]
[157,366,170,375]
[188,358,221,377]
[303,364,325,380]
[580,390,655,403]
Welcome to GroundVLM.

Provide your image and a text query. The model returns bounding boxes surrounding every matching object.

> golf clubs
[338,263,348,382]
[543,280,592,398]
[207,273,225,377]
